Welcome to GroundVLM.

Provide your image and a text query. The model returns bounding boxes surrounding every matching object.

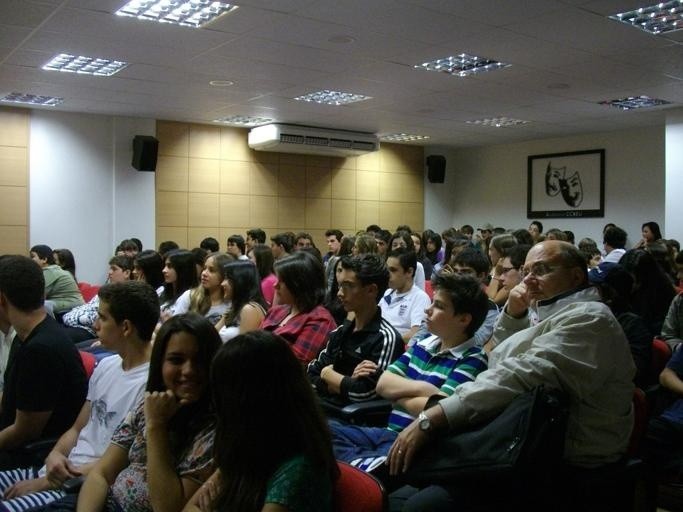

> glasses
[500,267,514,274]
[518,263,559,278]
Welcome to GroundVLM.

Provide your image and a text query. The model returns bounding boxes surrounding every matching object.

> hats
[477,223,493,233]
[587,261,634,296]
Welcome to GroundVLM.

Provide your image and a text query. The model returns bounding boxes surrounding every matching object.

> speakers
[427,155,446,184]
[132,135,160,173]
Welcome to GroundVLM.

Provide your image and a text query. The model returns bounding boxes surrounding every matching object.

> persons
[1,219,683,510]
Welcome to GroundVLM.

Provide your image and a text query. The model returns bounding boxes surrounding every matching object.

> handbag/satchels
[383,385,568,484]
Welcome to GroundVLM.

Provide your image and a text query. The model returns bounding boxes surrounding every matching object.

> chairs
[335,459,390,512]
[79,350,97,381]
